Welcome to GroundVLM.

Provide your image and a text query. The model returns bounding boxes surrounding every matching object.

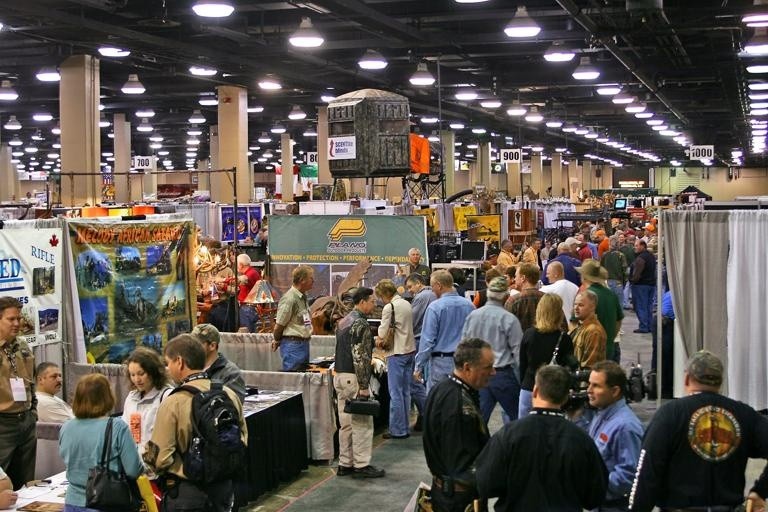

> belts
[434,478,465,492]
[0,413,25,419]
[432,352,453,356]
[282,335,313,342]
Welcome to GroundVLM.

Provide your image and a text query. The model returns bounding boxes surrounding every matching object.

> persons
[0,297,38,508]
[272,219,768,512]
[32,323,246,512]
[195,214,268,334]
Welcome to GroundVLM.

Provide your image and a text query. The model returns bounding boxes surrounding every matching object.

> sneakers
[383,433,409,439]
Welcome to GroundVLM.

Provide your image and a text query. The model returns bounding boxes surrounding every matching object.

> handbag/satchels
[375,328,394,350]
[85,466,141,512]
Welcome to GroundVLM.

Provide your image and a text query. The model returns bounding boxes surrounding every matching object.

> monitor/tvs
[614,199,627,209]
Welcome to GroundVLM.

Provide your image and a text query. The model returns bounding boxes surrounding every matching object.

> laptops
[450,241,487,265]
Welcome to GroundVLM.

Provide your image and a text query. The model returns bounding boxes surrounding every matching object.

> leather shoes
[337,465,353,476]
[353,466,384,477]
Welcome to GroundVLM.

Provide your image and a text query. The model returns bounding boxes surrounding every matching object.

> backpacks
[169,380,248,484]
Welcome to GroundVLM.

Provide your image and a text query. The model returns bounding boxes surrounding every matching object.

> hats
[488,277,507,292]
[191,324,220,343]
[574,259,608,282]
[687,350,723,385]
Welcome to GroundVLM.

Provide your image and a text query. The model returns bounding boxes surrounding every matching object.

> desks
[233,389,308,510]
[0,466,66,510]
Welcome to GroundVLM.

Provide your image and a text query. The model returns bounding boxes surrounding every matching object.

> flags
[274,165,299,199]
[409,133,430,176]
[298,165,318,197]
